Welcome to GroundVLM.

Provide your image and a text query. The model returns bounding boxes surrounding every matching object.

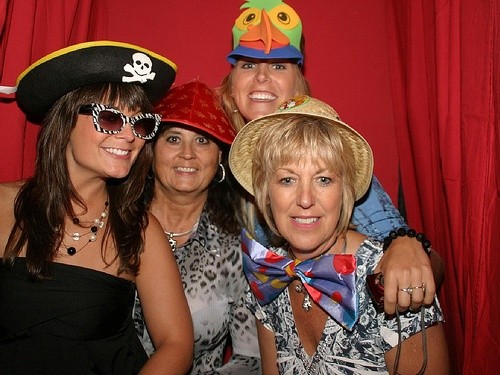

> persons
[132,0,447,375]
[0,41,194,375]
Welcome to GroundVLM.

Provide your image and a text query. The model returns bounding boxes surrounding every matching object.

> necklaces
[295,237,347,313]
[165,224,195,251]
[63,194,109,255]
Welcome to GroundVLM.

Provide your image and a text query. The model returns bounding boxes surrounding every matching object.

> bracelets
[383,228,431,255]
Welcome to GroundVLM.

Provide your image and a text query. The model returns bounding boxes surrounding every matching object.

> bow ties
[240,228,358,333]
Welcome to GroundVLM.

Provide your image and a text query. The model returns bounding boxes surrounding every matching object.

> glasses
[78,102,162,140]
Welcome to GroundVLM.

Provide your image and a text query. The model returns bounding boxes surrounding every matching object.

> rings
[402,288,413,294]
[416,283,425,292]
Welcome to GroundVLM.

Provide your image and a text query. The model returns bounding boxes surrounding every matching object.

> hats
[155,80,238,145]
[16,40,178,123]
[226,0,308,66]
[228,96,373,202]
